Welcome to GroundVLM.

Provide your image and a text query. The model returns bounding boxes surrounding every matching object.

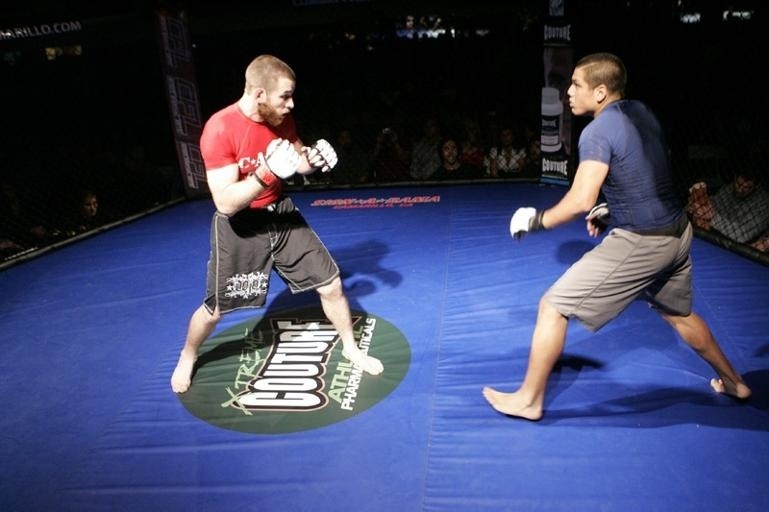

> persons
[659,116,769,253]
[168,54,386,395]
[294,110,542,187]
[481,51,753,422]
[2,140,186,256]
